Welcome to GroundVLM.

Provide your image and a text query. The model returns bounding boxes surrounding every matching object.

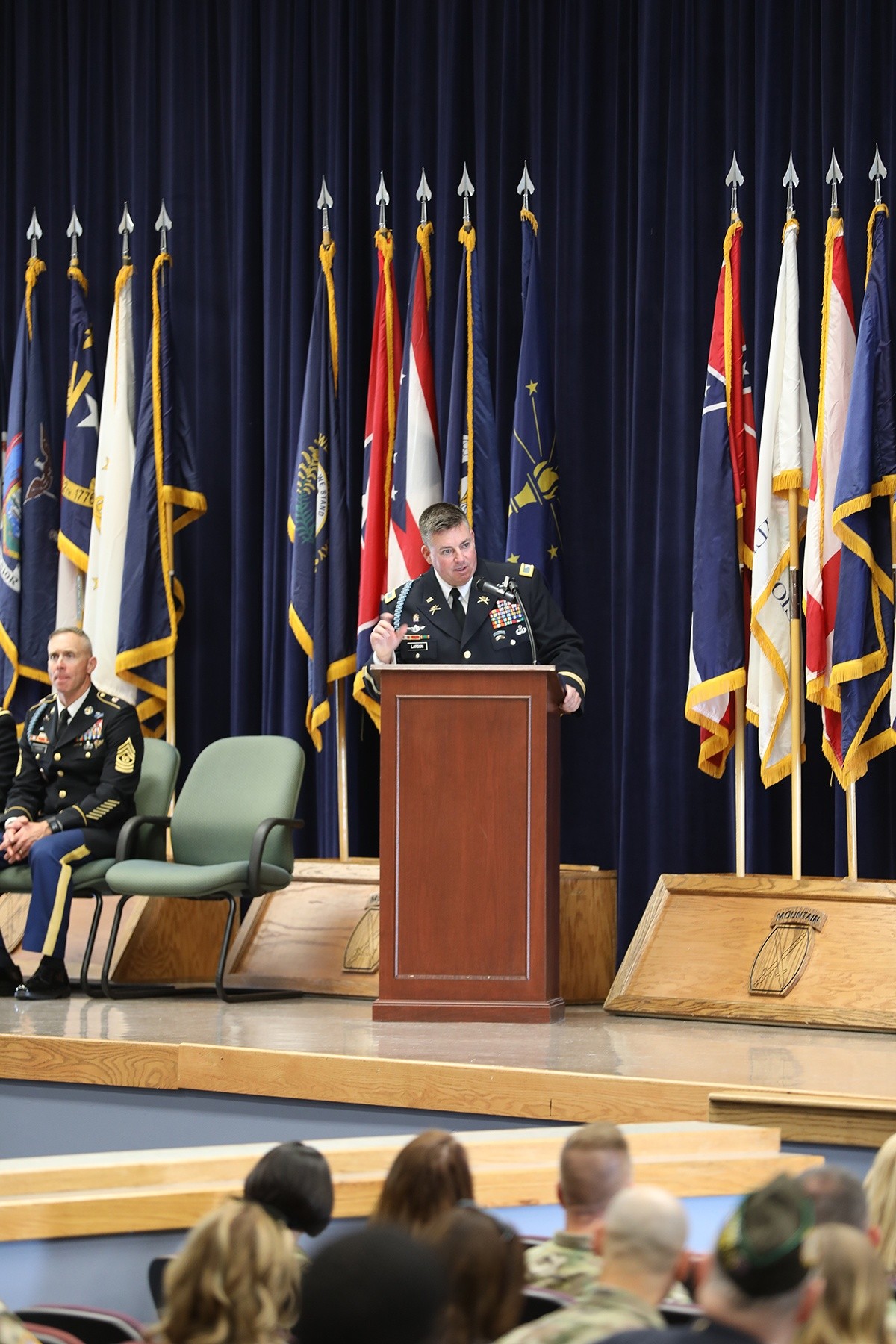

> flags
[284,206,563,756]
[0,252,210,741]
[684,204,896,789]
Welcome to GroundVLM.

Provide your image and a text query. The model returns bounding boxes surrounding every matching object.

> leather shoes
[0,965,24,997]
[14,966,71,1000]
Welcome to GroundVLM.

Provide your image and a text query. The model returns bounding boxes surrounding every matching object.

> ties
[55,709,71,741]
[449,587,467,633]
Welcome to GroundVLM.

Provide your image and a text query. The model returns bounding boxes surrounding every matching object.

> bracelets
[47,816,62,833]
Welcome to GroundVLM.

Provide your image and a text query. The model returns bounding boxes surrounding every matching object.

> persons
[361,502,588,717]
[148,1119,896,1344]
[0,627,144,999]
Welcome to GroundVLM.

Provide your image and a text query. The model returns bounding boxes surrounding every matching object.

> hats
[718,1172,819,1297]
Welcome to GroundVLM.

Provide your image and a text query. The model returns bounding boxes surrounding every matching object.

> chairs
[0,734,180,998]
[12,1233,758,1344]
[100,736,305,1003]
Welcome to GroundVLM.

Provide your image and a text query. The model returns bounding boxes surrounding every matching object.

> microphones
[475,578,520,604]
